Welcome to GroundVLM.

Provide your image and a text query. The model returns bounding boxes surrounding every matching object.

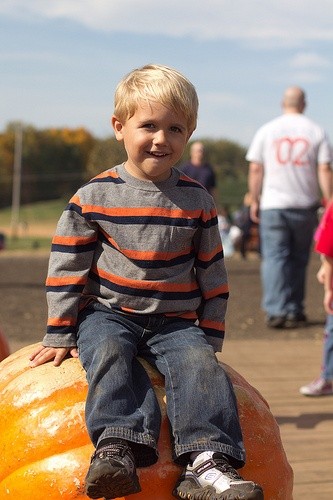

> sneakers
[299,376,333,396]
[171,451,264,500]
[84,439,142,500]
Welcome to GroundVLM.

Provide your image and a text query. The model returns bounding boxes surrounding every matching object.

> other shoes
[287,314,307,328]
[267,316,284,329]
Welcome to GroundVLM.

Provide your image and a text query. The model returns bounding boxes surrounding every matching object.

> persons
[180,85,333,396]
[30,66,264,500]
